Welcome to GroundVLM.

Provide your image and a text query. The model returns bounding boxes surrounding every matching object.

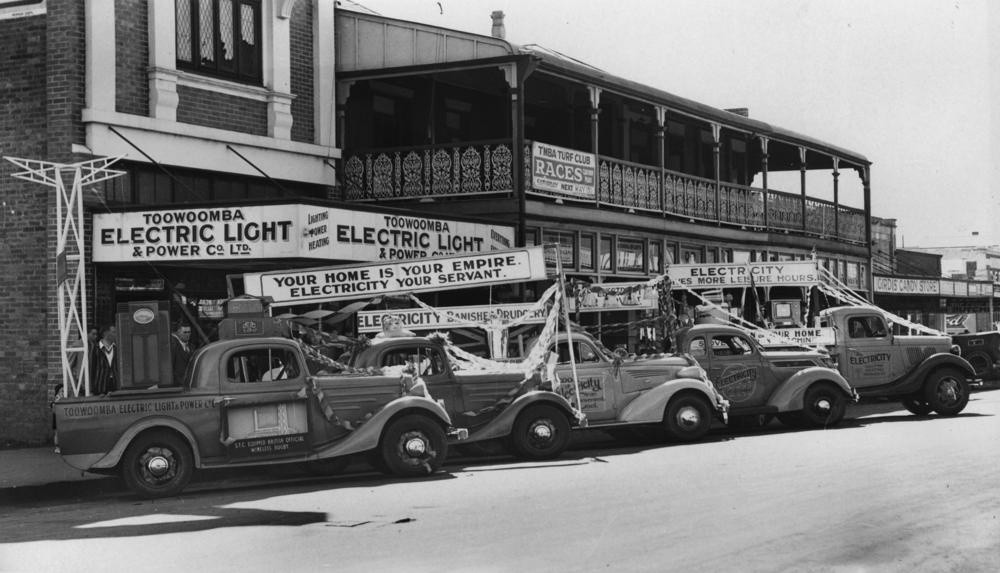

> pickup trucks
[628,324,859,426]
[55,335,467,500]
[780,306,978,417]
[313,337,587,463]
[441,333,728,443]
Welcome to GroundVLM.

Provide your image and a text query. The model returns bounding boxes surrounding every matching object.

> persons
[169,319,183,338]
[63,323,98,396]
[50,384,66,453]
[173,319,194,389]
[97,324,121,395]
